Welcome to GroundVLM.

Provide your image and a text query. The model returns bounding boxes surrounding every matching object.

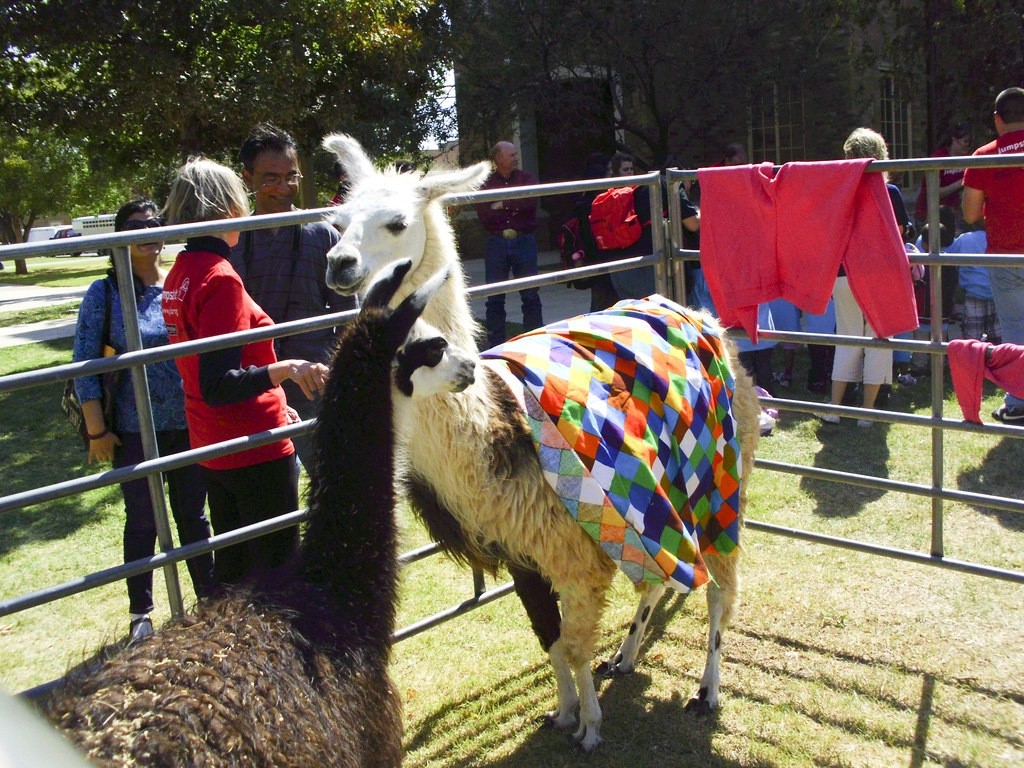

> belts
[489,228,533,240]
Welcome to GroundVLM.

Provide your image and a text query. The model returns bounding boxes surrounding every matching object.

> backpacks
[589,185,667,251]
[557,215,599,290]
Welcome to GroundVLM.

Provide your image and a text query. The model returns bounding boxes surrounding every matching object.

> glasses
[252,168,304,187]
[122,216,163,231]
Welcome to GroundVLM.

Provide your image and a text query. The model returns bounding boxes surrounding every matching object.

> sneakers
[806,378,827,394]
[992,403,1024,425]
[130,615,154,637]
[897,373,917,385]
[856,419,874,428]
[777,373,792,388]
[813,413,840,424]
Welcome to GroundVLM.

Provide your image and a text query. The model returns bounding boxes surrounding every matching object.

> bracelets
[87,428,108,440]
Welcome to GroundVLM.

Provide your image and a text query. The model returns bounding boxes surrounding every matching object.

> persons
[691,267,858,403]
[893,205,1000,385]
[228,123,358,475]
[812,127,910,427]
[577,153,617,311]
[72,198,214,641]
[607,157,701,299]
[162,157,328,594]
[475,141,543,345]
[914,125,971,234]
[960,87,1024,424]
[331,160,352,206]
[710,142,745,167]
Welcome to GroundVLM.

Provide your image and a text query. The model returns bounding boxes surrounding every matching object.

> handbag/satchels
[60,280,121,452]
